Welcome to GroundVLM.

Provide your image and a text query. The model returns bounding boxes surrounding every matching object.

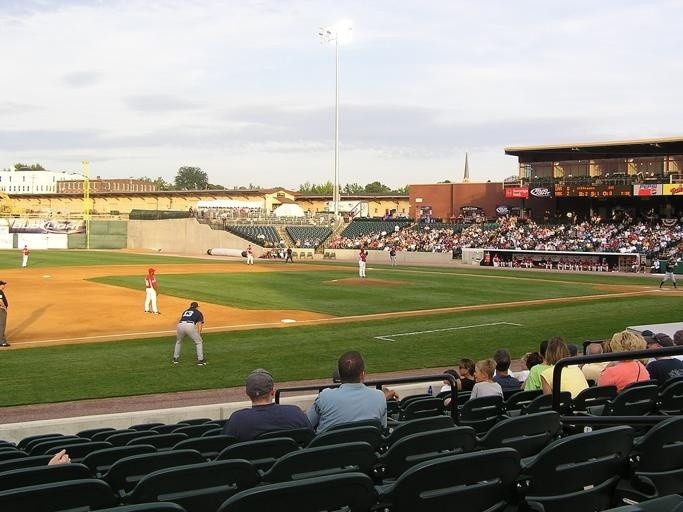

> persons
[439,329,682,418]
[143,268,162,315]
[21,245,29,268]
[331,369,400,401]
[304,351,388,438]
[190,175,683,289]
[47,449,72,465]
[223,369,315,445]
[0,280,10,347]
[173,302,209,366]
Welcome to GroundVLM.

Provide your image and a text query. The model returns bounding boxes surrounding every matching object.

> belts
[179,322,193,323]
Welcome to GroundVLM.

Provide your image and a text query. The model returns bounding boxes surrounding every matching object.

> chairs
[227,222,470,250]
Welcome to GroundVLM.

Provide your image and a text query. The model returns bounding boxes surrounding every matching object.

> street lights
[80,157,94,252]
[318,26,338,214]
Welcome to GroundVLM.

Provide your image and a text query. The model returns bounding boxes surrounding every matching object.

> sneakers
[197,359,210,366]
[0,343,10,347]
[153,312,160,314]
[172,358,179,364]
[145,311,151,313]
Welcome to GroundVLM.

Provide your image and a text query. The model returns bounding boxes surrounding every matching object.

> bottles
[427,386,433,396]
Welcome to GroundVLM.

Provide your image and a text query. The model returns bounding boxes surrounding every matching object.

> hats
[246,369,273,395]
[0,281,7,285]
[149,269,156,273]
[191,302,198,307]
[643,333,674,347]
